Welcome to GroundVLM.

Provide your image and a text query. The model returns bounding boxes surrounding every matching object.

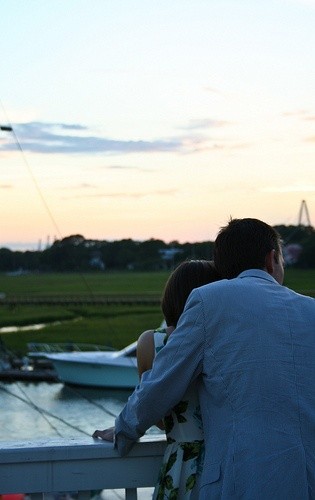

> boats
[28,337,150,389]
[0,343,116,383]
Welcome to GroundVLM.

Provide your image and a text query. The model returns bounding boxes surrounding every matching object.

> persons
[92,219,315,499]
[137,261,217,499]
